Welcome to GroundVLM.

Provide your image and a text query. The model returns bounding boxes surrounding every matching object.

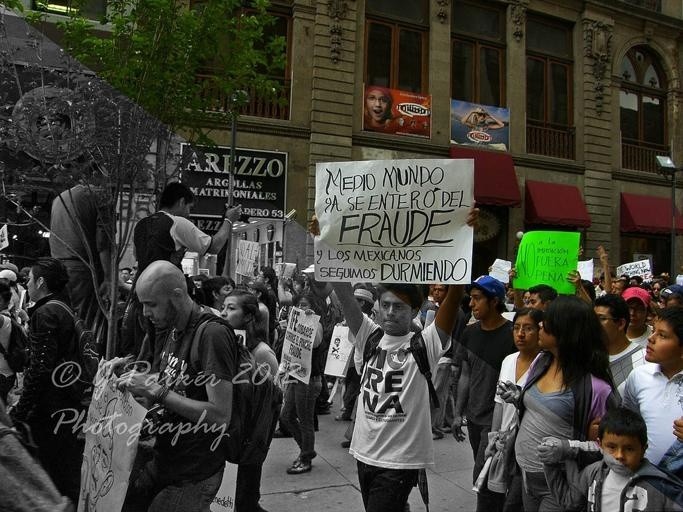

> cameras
[222,207,249,224]
[497,380,511,393]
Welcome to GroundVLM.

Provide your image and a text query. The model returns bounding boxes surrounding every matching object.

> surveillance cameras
[283,208,297,221]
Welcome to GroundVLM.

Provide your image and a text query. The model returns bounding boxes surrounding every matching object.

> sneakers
[286,449,319,475]
[430,413,470,441]
[271,380,353,449]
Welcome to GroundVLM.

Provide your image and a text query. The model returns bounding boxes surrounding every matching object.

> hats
[243,281,268,295]
[660,283,683,299]
[300,263,314,274]
[352,288,375,305]
[0,262,19,276]
[620,286,651,309]
[0,269,17,282]
[468,274,507,303]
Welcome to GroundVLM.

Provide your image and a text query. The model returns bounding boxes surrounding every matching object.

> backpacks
[0,421,76,512]
[0,311,31,374]
[44,299,102,404]
[13,308,31,337]
[163,311,285,468]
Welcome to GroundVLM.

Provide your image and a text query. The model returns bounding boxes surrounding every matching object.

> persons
[364,86,405,133]
[461,107,504,144]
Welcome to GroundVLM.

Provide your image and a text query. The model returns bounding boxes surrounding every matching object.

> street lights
[655,153,682,284]
[225,88,249,279]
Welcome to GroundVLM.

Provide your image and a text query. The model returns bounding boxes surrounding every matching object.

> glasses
[369,310,380,318]
